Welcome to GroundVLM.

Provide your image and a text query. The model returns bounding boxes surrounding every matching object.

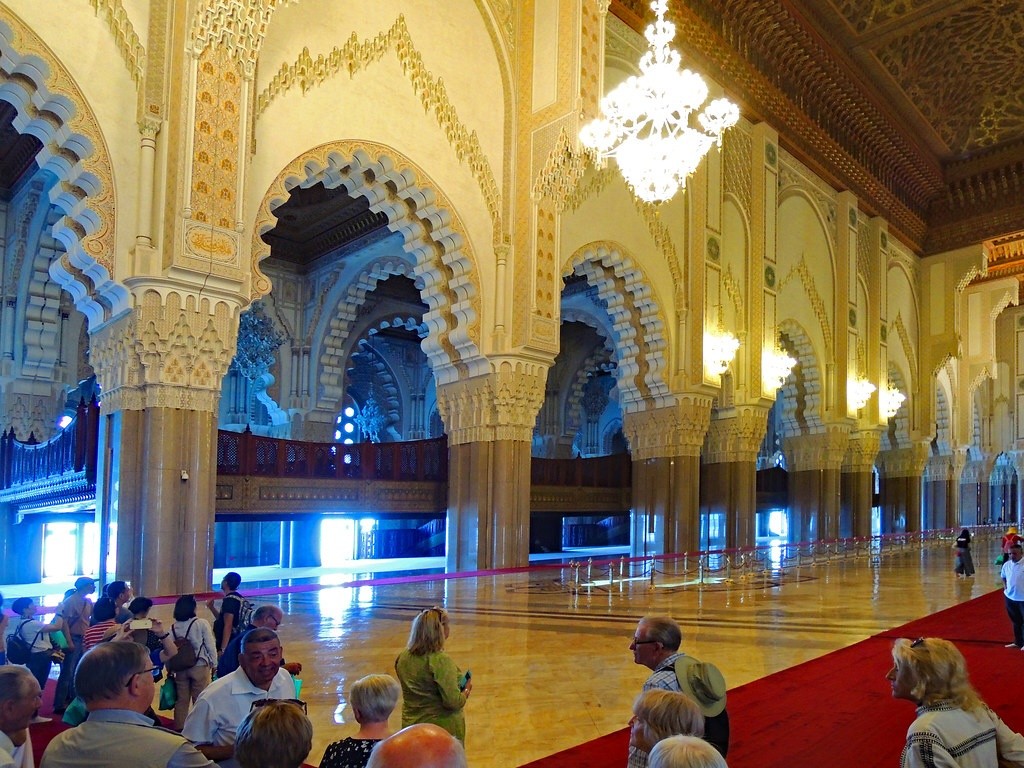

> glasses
[633,637,660,645]
[270,615,280,626]
[1008,552,1020,555]
[123,665,162,688]
[421,608,443,623]
[248,698,308,717]
[910,637,923,649]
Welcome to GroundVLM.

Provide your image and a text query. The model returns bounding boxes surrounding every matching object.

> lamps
[234,299,290,382]
[880,368,905,416]
[574,0,742,214]
[703,302,742,378]
[844,336,877,412]
[353,332,396,445]
[761,326,798,395]
[581,382,611,426]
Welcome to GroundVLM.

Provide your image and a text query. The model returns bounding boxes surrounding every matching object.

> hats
[95,623,131,641]
[75,576,101,589]
[673,654,728,717]
[1005,527,1018,535]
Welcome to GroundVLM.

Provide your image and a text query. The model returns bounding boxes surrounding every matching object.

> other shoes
[26,707,67,725]
[956,574,971,577]
[1004,642,1024,651]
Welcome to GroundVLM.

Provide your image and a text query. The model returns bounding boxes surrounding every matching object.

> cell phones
[125,580,130,589]
[129,619,153,630]
[458,669,472,692]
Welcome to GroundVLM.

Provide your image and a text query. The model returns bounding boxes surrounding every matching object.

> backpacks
[1003,535,1017,553]
[5,618,44,665]
[165,617,210,672]
[223,594,255,632]
[656,665,729,760]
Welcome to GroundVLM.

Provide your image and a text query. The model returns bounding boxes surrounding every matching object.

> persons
[179,628,296,767]
[38,641,222,767]
[648,735,727,768]
[394,606,472,750]
[629,688,705,754]
[0,571,302,733]
[886,637,1024,768]
[627,614,728,768]
[1000,544,1024,651]
[1,665,43,768]
[365,723,467,768]
[1001,527,1024,565]
[234,702,313,767]
[319,675,401,768]
[953,529,976,577]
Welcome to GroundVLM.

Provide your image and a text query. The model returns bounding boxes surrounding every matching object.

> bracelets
[464,688,470,696]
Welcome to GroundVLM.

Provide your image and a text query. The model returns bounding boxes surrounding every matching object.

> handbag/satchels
[158,673,178,711]
[994,553,1004,565]
[62,696,87,726]
[955,550,961,557]
[49,613,69,650]
[291,675,302,700]
